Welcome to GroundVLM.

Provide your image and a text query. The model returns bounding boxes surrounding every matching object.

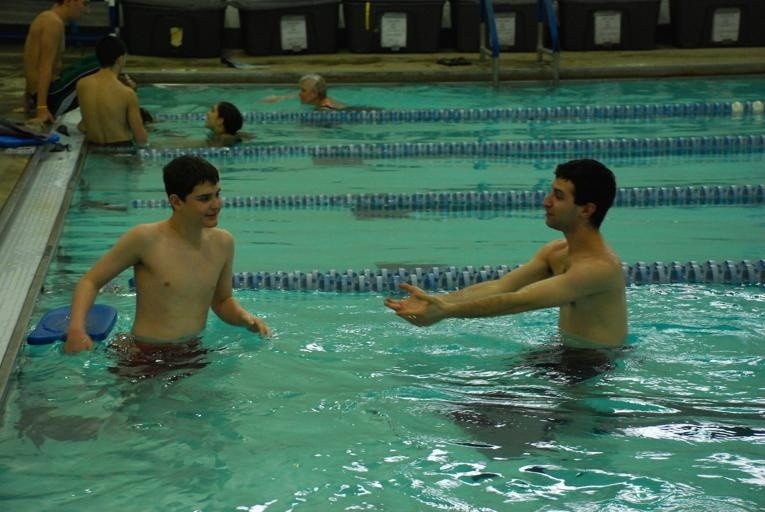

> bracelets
[33,103,50,113]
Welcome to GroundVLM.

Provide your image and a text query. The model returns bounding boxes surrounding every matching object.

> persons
[447,357,630,459]
[61,155,279,359]
[189,101,256,147]
[261,73,354,112]
[24,1,157,127]
[75,35,147,153]
[383,159,627,352]
[18,365,229,447]
